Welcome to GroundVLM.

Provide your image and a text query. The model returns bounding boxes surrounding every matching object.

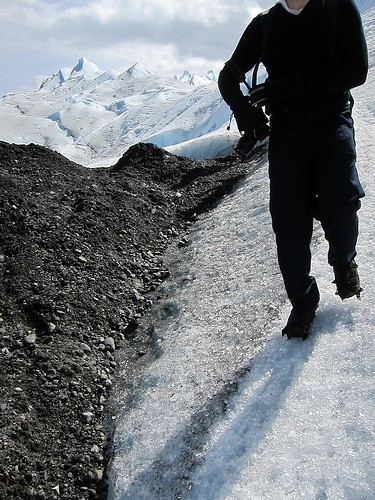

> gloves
[231,95,269,131]
[266,81,303,116]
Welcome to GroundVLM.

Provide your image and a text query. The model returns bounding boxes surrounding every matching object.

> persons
[215,0,369,340]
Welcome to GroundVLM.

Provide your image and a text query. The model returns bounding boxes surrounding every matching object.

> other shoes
[332,258,363,301]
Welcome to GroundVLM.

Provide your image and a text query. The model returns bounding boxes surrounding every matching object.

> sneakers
[282,278,319,343]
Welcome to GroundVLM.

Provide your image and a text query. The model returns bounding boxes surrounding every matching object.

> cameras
[234,122,272,158]
